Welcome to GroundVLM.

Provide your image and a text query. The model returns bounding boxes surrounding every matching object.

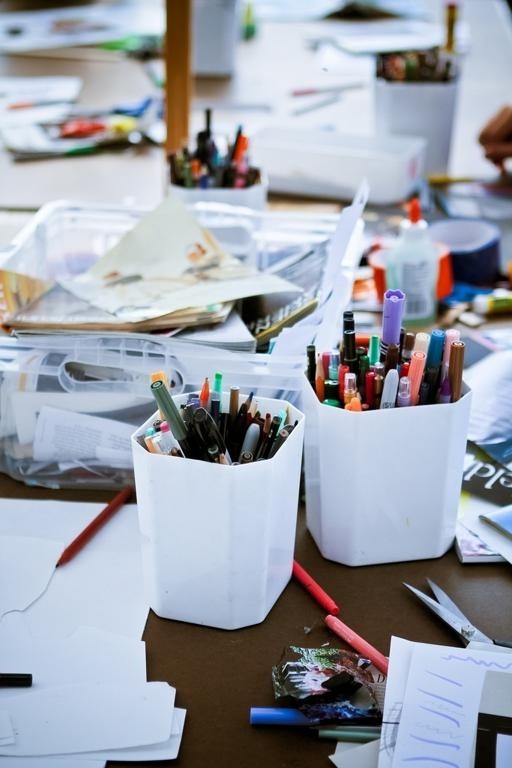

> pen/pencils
[55,483,133,569]
[136,370,298,467]
[324,614,389,675]
[293,560,340,615]
[309,724,381,733]
[166,108,260,189]
[378,3,460,82]
[318,729,381,743]
[6,98,76,109]
[246,705,383,730]
[306,287,466,411]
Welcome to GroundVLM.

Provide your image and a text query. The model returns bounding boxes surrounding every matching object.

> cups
[190,0,236,78]
[428,217,501,283]
[168,166,268,207]
[372,67,460,174]
[367,240,454,301]
[299,363,471,567]
[130,389,307,629]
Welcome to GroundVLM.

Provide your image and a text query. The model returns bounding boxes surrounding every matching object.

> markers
[0,674,34,692]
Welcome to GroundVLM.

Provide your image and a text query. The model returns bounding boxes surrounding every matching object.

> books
[454,443,510,564]
[3,238,340,351]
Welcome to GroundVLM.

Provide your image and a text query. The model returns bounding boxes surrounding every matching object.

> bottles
[394,197,436,324]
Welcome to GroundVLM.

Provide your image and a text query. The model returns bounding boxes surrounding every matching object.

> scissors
[402,576,512,648]
